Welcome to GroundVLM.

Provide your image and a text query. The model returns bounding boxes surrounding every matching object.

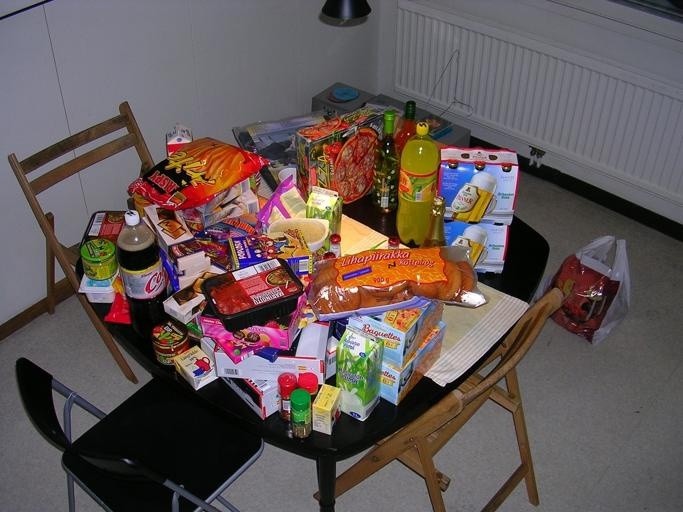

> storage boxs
[367,92,471,148]
[311,81,377,115]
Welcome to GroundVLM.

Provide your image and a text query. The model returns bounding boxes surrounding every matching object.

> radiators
[393,0,683,209]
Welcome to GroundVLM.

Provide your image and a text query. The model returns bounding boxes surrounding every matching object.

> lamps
[321,0,372,21]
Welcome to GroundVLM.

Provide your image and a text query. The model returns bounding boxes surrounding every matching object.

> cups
[451,230,489,270]
[450,176,498,225]
[277,167,296,186]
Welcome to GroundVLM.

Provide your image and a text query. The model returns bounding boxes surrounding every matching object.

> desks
[74,183,549,512]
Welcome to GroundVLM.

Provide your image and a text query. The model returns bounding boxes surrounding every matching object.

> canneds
[150,320,190,369]
[81,238,117,281]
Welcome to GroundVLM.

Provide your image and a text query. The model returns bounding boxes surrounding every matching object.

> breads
[310,248,476,316]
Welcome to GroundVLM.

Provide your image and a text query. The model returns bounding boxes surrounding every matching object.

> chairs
[13,353,264,512]
[8,100,157,383]
[314,287,563,512]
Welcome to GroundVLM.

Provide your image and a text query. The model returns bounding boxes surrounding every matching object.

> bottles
[116,211,171,335]
[321,235,343,261]
[279,373,320,439]
[373,101,448,249]
